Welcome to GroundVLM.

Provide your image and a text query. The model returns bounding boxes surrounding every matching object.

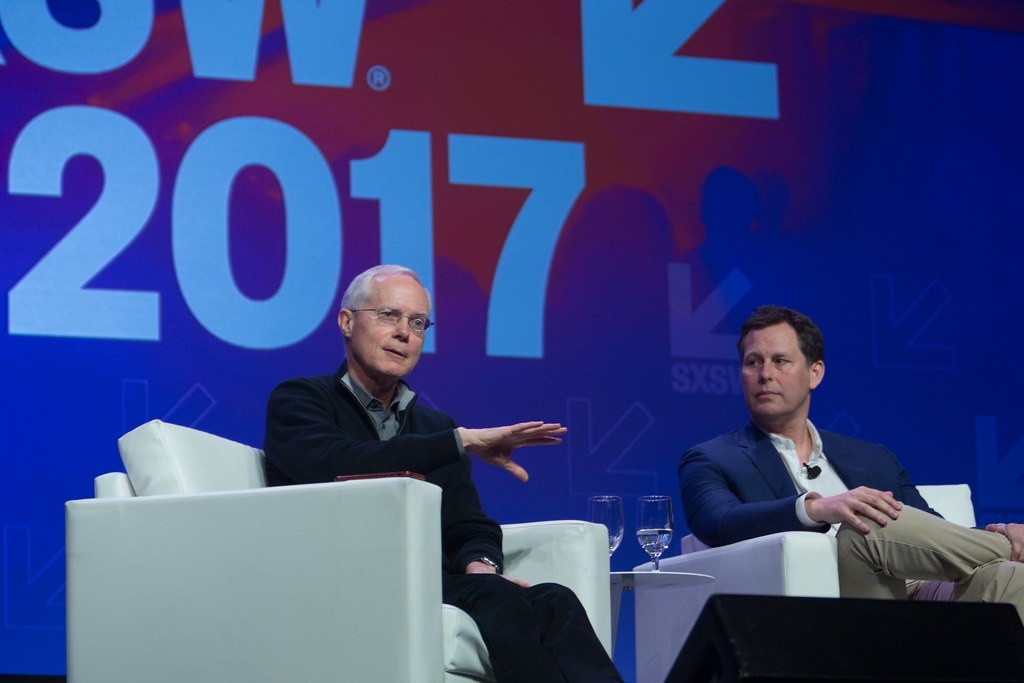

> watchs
[465,555,501,574]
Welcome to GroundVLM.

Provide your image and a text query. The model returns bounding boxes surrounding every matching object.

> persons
[259,264,624,677]
[680,309,1023,619]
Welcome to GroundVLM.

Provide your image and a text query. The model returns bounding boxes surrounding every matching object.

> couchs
[632,483,976,683]
[65,420,611,683]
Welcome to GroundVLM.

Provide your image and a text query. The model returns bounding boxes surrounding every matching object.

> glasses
[347,306,437,334]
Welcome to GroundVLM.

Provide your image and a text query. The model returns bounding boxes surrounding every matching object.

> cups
[587,496,624,558]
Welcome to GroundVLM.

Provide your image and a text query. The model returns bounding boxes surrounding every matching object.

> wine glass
[634,495,674,572]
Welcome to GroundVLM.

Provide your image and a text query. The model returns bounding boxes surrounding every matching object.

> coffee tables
[609,572,715,662]
